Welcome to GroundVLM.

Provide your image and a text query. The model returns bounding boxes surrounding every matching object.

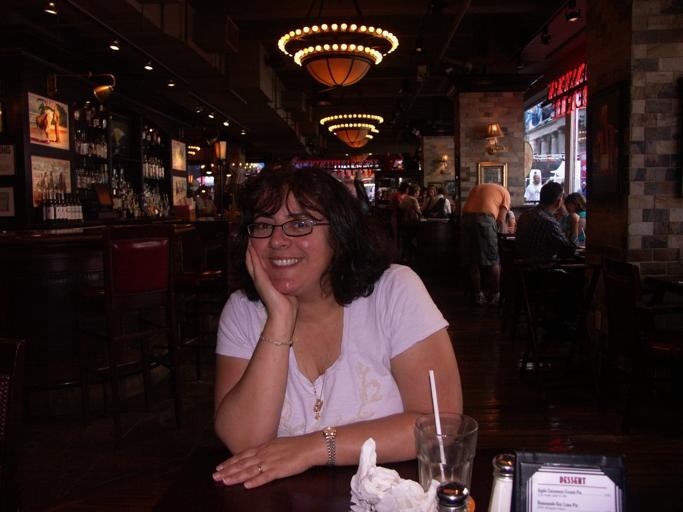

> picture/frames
[476,160,509,192]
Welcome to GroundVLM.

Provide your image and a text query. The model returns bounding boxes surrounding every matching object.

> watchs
[322,426,336,467]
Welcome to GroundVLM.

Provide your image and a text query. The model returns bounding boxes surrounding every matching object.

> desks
[154,440,620,512]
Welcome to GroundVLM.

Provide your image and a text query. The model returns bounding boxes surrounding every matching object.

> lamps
[184,88,255,137]
[268,11,402,90]
[316,108,386,151]
[483,122,506,157]
[214,139,229,218]
[440,152,450,171]
[42,0,186,88]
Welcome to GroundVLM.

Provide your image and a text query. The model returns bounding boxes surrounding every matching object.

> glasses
[247,219,330,238]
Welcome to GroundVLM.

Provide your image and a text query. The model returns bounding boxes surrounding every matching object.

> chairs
[1,331,35,511]
[69,219,186,441]
[596,257,682,432]
[498,232,546,358]
[175,218,242,390]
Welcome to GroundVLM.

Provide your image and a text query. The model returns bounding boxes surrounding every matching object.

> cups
[414,413,478,493]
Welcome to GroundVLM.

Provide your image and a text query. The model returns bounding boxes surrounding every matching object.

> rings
[257,463,263,474]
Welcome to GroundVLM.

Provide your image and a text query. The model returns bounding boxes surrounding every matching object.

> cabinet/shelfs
[72,100,112,211]
[140,121,172,214]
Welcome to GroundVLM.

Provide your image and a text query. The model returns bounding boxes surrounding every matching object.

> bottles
[73,101,106,129]
[143,191,169,216]
[487,454,513,511]
[142,153,164,179]
[436,480,468,512]
[73,129,107,159]
[37,188,83,228]
[142,125,160,144]
[110,168,140,220]
[73,168,102,190]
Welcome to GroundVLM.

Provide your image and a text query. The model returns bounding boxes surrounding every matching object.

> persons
[388,175,588,341]
[236,132,300,285]
[196,187,217,216]
[211,166,465,490]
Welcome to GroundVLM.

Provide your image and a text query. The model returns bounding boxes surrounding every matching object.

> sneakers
[477,296,504,303]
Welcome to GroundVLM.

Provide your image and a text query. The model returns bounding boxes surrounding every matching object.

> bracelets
[260,334,293,347]
